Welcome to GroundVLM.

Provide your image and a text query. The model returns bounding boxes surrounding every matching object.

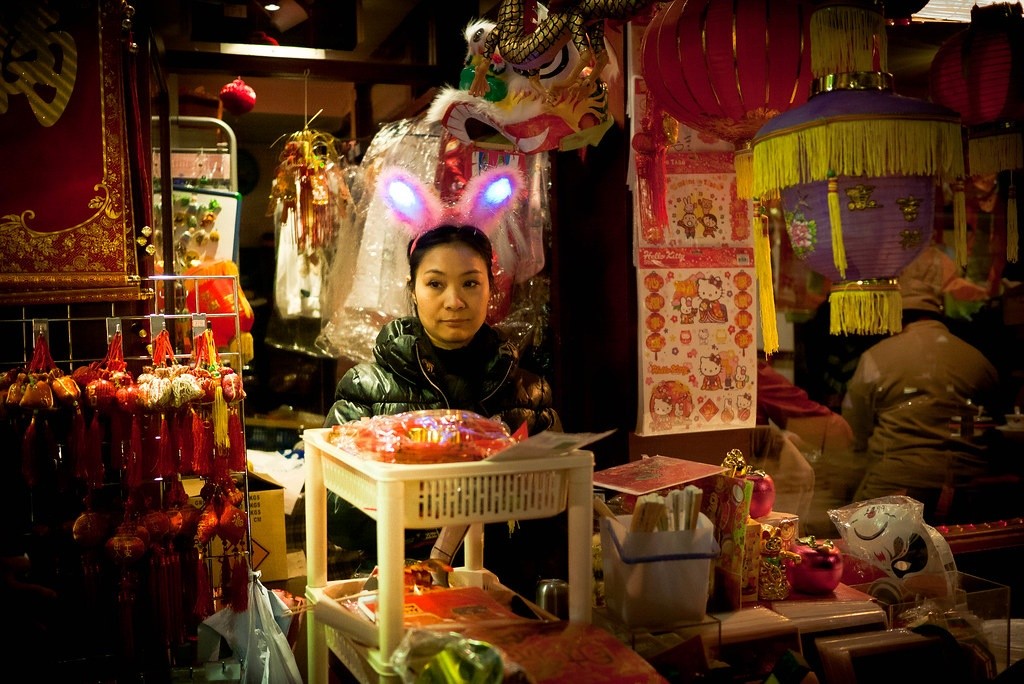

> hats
[895,246,957,315]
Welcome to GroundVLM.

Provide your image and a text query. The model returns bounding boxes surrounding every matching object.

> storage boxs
[847,570,1012,670]
[208,488,290,585]
[601,513,714,627]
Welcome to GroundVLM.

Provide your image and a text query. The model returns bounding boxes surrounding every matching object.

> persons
[323,226,564,684]
[757,256,1024,525]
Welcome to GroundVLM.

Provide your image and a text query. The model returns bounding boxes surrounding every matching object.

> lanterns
[219,78,256,118]
[182,262,251,374]
[640,1,1024,361]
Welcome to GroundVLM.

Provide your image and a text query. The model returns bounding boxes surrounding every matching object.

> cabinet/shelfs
[302,428,592,684]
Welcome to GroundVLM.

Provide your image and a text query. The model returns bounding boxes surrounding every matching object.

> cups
[1005,415,1024,430]
